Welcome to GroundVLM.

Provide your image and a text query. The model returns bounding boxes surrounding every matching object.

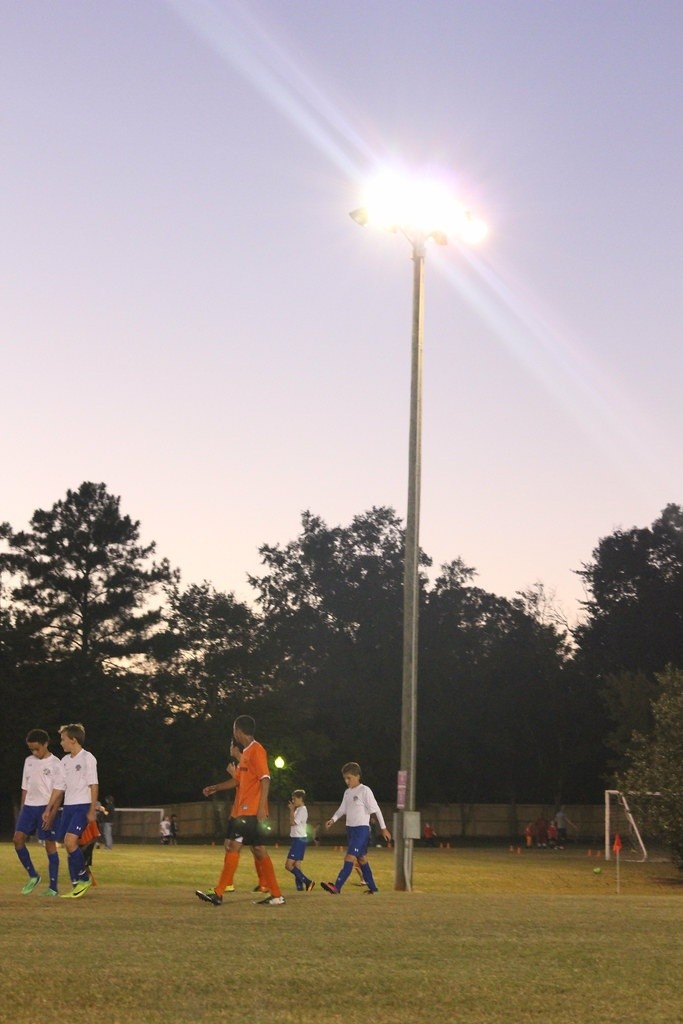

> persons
[10,729,63,898]
[195,715,286,906]
[369,816,439,848]
[42,723,99,899]
[546,805,579,851]
[320,762,393,895]
[285,789,316,892]
[159,813,178,845]
[523,821,534,849]
[94,796,116,851]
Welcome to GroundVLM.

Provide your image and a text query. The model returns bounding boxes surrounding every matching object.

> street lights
[343,172,476,891]
[275,754,286,849]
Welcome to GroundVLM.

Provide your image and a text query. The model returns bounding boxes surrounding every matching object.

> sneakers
[21,875,41,895]
[255,896,285,905]
[250,885,270,895]
[62,879,92,898]
[196,888,222,906]
[42,888,57,898]
[321,881,340,894]
[306,880,315,891]
[205,884,234,895]
[362,888,378,895]
